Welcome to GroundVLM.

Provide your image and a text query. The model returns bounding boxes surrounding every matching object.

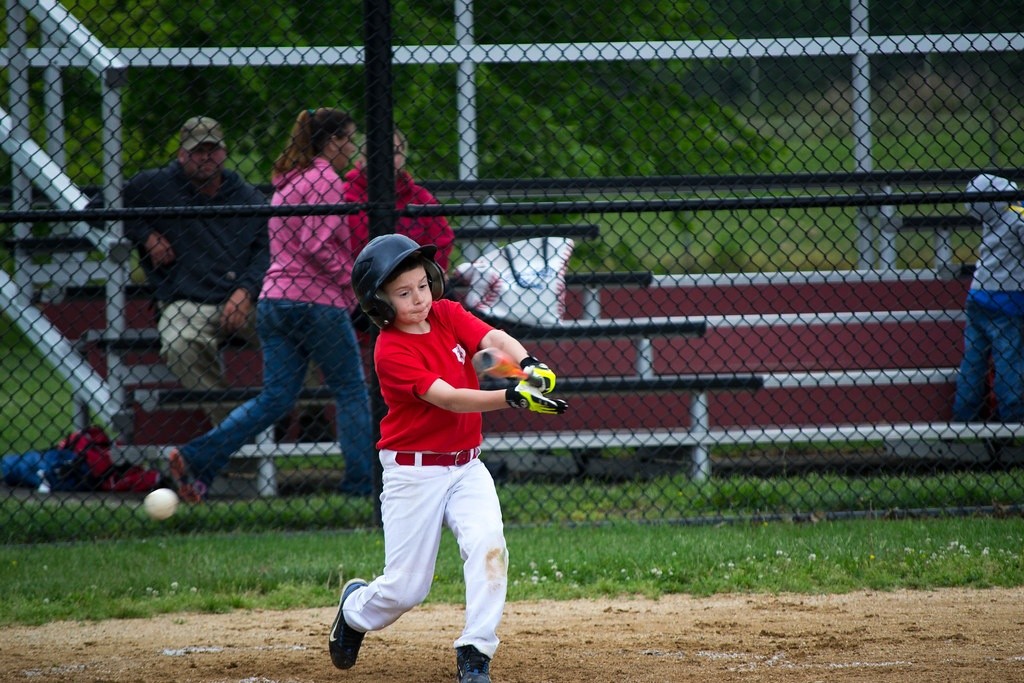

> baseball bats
[472,347,542,388]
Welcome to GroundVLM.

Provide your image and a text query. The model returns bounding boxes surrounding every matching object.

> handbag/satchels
[453,237,575,328]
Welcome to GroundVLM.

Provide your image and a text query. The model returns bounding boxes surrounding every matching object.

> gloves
[505,377,568,414]
[505,353,556,395]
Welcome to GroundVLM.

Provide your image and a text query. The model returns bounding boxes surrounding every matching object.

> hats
[180,116,226,151]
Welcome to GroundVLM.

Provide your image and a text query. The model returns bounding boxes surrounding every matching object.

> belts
[395,448,479,467]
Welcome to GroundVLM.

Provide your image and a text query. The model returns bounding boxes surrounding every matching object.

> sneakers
[329,578,367,670]
[456,645,491,683]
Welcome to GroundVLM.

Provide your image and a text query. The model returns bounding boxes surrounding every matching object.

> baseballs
[145,488,177,519]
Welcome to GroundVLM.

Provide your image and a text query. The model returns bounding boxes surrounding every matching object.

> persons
[328,233,569,683]
[948,175,1024,425]
[338,124,455,425]
[167,108,378,503]
[85,116,333,442]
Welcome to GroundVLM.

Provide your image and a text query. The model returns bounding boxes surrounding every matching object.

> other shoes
[227,459,278,479]
[159,446,206,504]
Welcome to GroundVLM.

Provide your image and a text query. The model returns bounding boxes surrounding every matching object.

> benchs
[86,259,1021,463]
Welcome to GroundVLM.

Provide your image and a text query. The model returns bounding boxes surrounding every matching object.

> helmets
[352,233,445,328]
